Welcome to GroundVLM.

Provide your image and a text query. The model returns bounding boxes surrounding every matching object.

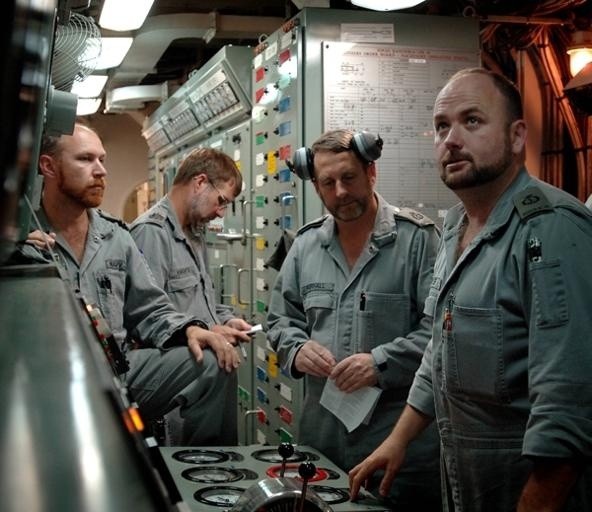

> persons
[265,132,447,509]
[348,65,588,511]
[132,148,253,443]
[29,122,240,445]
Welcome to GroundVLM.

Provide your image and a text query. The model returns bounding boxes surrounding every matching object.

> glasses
[208,182,229,210]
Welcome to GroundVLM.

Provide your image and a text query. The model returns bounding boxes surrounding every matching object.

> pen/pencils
[360,293,365,311]
[105,275,112,294]
[238,341,248,362]
[444,307,452,336]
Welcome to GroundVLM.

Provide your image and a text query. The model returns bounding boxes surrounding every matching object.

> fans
[49,10,103,92]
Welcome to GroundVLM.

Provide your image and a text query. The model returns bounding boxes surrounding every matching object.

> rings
[225,341,230,344]
